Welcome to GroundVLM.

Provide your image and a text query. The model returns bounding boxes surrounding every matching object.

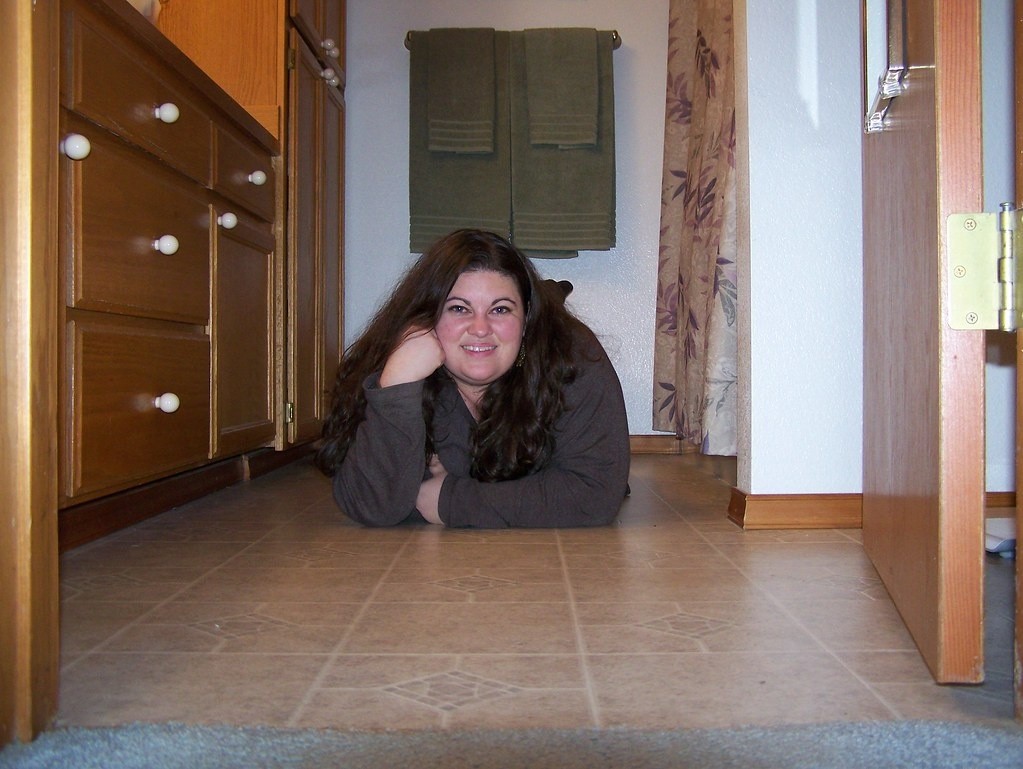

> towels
[523,27,598,150]
[404,28,512,253]
[510,27,622,260]
[428,27,495,155]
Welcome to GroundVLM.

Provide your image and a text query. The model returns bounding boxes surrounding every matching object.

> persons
[316,229,630,529]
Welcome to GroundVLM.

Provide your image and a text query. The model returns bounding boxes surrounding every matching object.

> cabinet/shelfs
[45,0,348,559]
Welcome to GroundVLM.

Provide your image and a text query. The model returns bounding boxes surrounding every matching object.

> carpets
[0,719,1023,769]
